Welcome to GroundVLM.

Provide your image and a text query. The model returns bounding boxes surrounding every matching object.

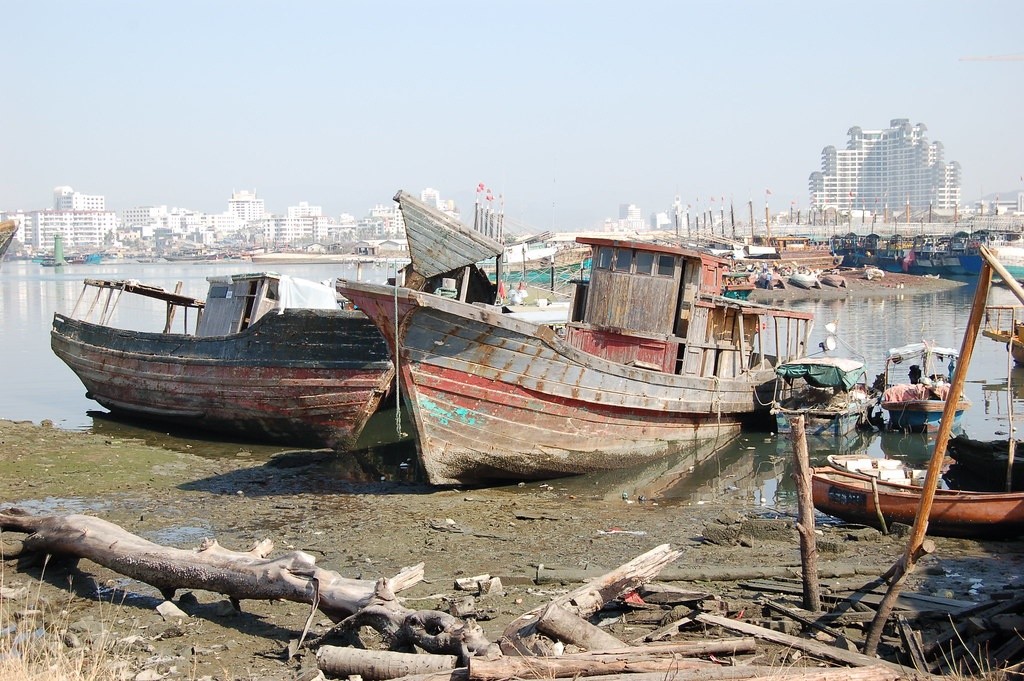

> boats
[862,268,885,282]
[474,230,563,267]
[162,251,230,262]
[823,275,847,287]
[982,305,1024,365]
[759,274,787,290]
[830,230,1024,279]
[775,422,874,512]
[878,343,970,434]
[788,276,822,289]
[720,272,753,300]
[881,423,962,467]
[48,270,397,452]
[336,234,814,486]
[31,250,105,265]
[808,451,1024,533]
[947,435,1024,476]
[770,352,878,435]
[0,219,21,271]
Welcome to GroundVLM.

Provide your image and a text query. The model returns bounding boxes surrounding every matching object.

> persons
[760,260,767,273]
[764,273,771,289]
